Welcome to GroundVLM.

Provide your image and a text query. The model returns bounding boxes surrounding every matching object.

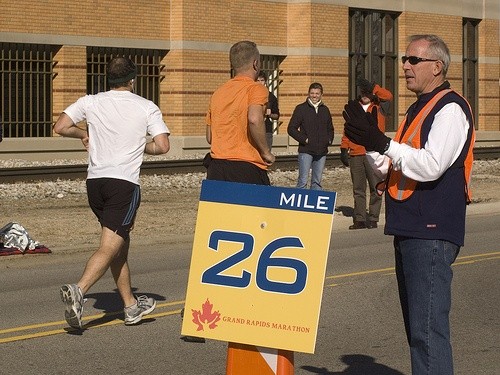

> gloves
[357,79,375,94]
[340,148,349,166]
[342,100,391,155]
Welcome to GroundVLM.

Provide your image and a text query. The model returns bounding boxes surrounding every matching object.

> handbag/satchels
[0,221,41,254]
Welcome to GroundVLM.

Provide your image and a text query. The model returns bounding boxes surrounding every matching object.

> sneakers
[123,294,157,325]
[59,283,88,330]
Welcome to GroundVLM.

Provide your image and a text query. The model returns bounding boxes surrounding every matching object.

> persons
[340,79,394,230]
[203,41,281,186]
[287,83,335,190]
[53,56,170,330]
[342,33,475,375]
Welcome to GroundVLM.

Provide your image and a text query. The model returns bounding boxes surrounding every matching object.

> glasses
[402,56,436,65]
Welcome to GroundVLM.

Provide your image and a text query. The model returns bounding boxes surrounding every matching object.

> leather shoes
[349,221,367,229]
[367,221,377,229]
